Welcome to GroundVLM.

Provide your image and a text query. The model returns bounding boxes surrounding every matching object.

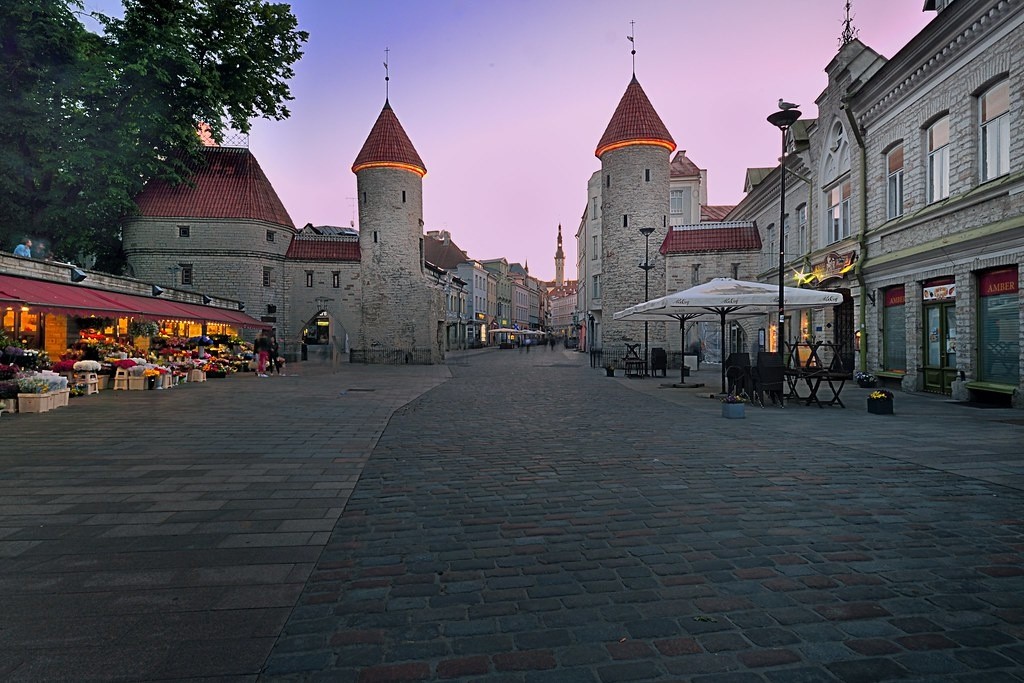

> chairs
[651,348,667,377]
[726,351,783,407]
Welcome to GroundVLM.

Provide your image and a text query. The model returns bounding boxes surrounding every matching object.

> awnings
[0,272,274,331]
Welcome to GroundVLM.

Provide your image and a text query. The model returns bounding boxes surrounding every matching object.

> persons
[257,332,269,378]
[254,332,261,376]
[302,335,308,361]
[269,336,283,376]
[518,333,571,355]
[13,238,32,258]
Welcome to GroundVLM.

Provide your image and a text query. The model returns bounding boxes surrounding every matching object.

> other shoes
[258,374,268,378]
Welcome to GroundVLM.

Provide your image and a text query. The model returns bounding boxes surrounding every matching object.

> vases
[722,403,745,418]
[0,342,282,414]
[867,398,893,415]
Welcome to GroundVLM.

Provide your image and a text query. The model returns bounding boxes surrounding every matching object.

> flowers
[0,329,87,398]
[51,341,171,376]
[865,389,894,399]
[154,330,284,383]
[720,392,749,403]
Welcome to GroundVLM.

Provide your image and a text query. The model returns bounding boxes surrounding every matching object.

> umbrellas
[490,328,546,345]
[612,276,844,400]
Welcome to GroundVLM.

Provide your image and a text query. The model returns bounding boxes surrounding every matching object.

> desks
[623,343,646,379]
[783,340,849,408]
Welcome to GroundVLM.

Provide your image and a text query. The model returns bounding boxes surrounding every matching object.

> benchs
[964,381,1019,409]
[874,371,907,390]
